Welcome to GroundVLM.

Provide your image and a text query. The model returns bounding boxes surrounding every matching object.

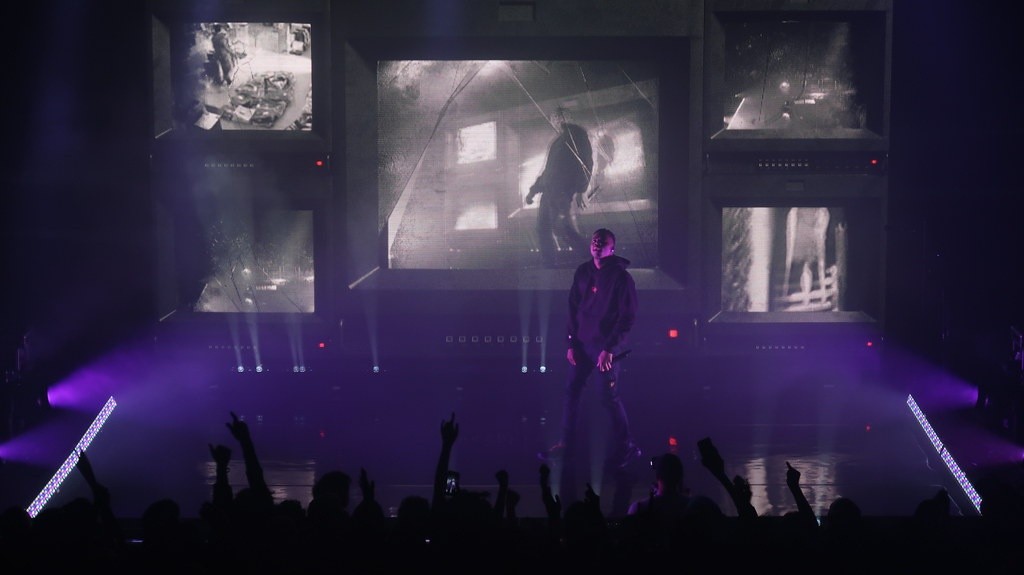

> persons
[212,25,235,86]
[525,106,594,266]
[1,412,1024,575]
[564,229,641,467]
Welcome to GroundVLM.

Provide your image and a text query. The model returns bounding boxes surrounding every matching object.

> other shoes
[607,445,642,468]
[537,441,578,461]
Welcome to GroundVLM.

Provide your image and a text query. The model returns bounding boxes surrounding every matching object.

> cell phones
[697,437,713,457]
[446,472,460,495]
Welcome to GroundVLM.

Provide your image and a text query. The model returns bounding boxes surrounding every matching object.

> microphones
[612,349,632,362]
